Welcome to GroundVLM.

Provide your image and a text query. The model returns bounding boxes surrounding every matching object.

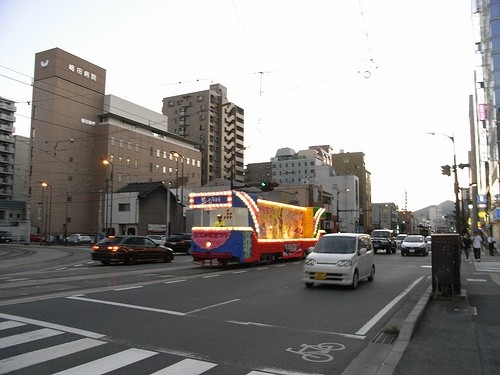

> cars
[147,235,166,246]
[31,233,48,242]
[64,233,91,244]
[401,235,428,256]
[91,236,174,265]
[426,236,431,250]
[164,233,192,255]
[302,233,376,289]
[0,231,13,243]
[397,234,408,247]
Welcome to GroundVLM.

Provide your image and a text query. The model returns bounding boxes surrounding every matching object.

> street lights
[336,188,350,233]
[169,151,185,233]
[101,158,113,235]
[42,182,52,242]
[426,132,460,234]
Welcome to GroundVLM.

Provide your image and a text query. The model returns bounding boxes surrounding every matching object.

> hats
[217,214,222,217]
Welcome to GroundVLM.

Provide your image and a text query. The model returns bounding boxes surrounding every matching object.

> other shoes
[475,259,481,262]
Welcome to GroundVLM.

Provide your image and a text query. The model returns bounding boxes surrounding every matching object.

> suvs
[371,229,397,254]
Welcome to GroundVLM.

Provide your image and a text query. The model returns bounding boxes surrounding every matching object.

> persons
[462,231,496,261]
[214,214,226,227]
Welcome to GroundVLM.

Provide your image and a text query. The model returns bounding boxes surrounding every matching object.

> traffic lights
[261,182,268,192]
[440,165,450,176]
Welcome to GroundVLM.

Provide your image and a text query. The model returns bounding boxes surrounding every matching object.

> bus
[188,190,327,269]
